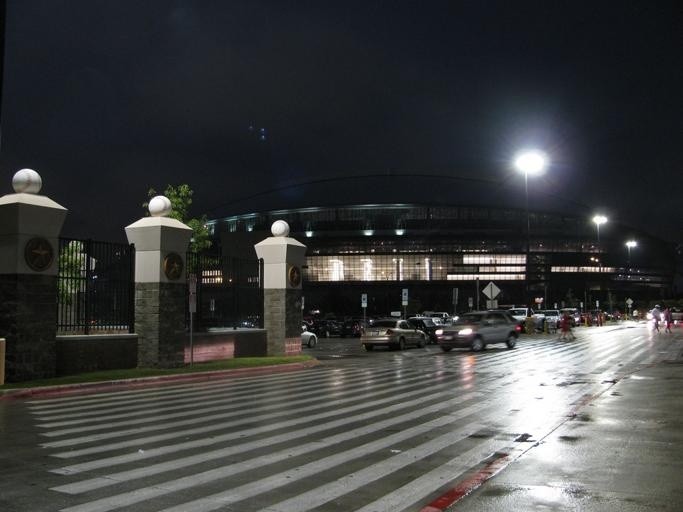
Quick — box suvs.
[434,311,521,353]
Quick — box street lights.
[626,240,637,321]
[516,146,547,336]
[593,215,608,325]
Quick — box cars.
[301,329,318,348]
[484,304,683,339]
[240,311,460,339]
[360,319,427,351]
[397,316,452,345]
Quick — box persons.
[663,306,672,333]
[558,312,570,341]
[650,304,661,334]
[562,312,576,339]
